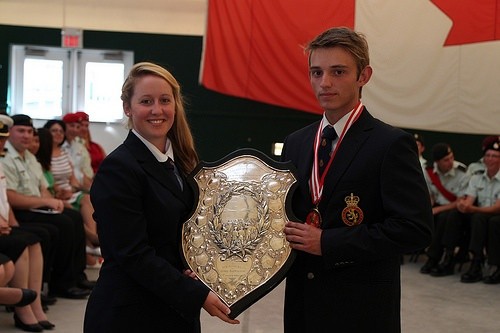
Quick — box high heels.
[13,311,56,332]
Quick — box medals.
[306,207,323,229]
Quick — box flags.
[198,0,500,137]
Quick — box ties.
[166,160,184,192]
[316,125,338,177]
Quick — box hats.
[63,113,81,123]
[431,142,453,161]
[413,133,423,143]
[0,115,14,136]
[484,139,500,152]
[76,111,89,122]
[9,114,34,127]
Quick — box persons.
[402,134,500,286]
[83,63,241,333]
[0,112,105,333]
[278,27,431,333]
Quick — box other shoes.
[461,262,484,282]
[86,256,104,268]
[484,267,500,285]
[42,295,57,312]
[420,253,442,273]
[0,288,37,308]
[50,279,97,298]
[432,251,455,277]
